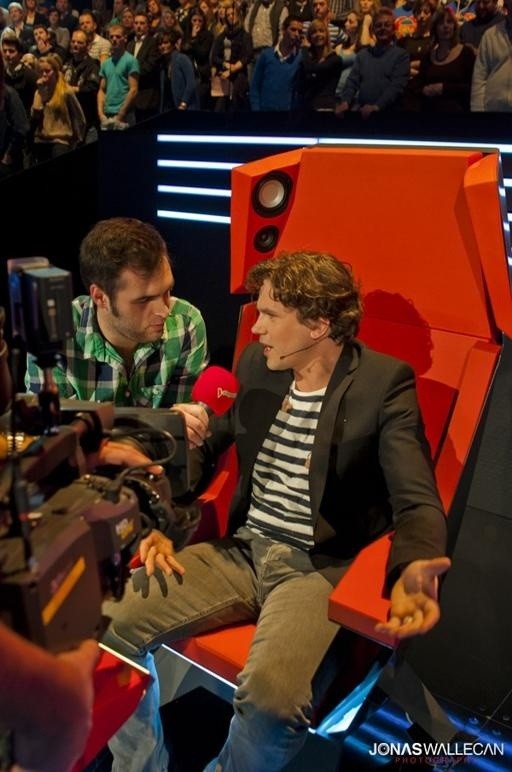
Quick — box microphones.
[280,332,333,359]
[189,364,242,419]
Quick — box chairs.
[68,144,512,771]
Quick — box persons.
[97,248,454,772]
[1,0,512,184]
[22,215,214,453]
